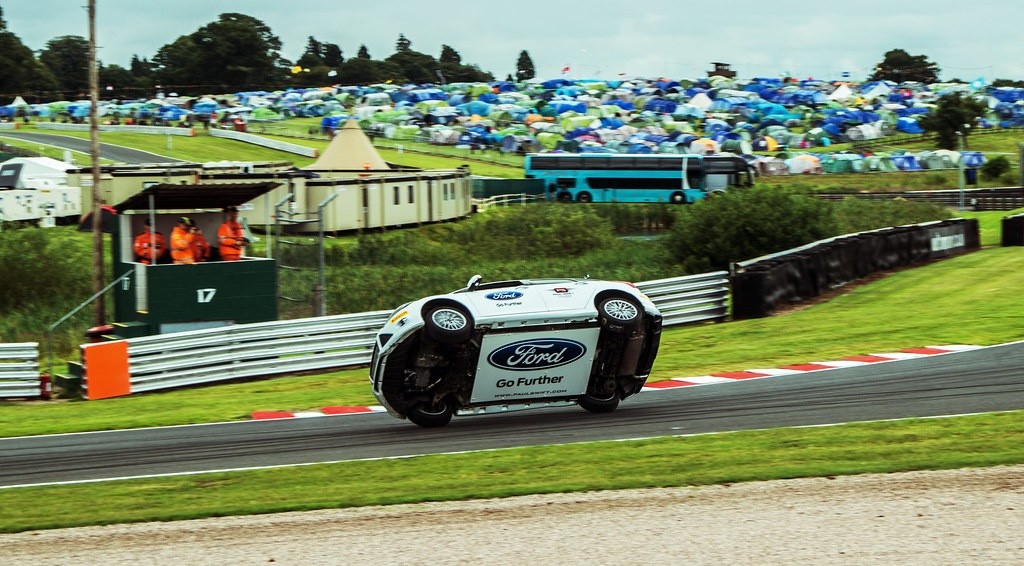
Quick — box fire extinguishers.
[40,368,52,399]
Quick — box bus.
[524,152,760,204]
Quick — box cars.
[368,273,663,429]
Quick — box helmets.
[177,217,196,227]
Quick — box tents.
[0,76,1023,181]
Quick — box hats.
[144,219,151,226]
[224,206,239,213]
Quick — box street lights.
[956,117,983,210]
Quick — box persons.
[216,207,251,261]
[964,164,979,189]
[133,220,167,264]
[170,217,211,264]
